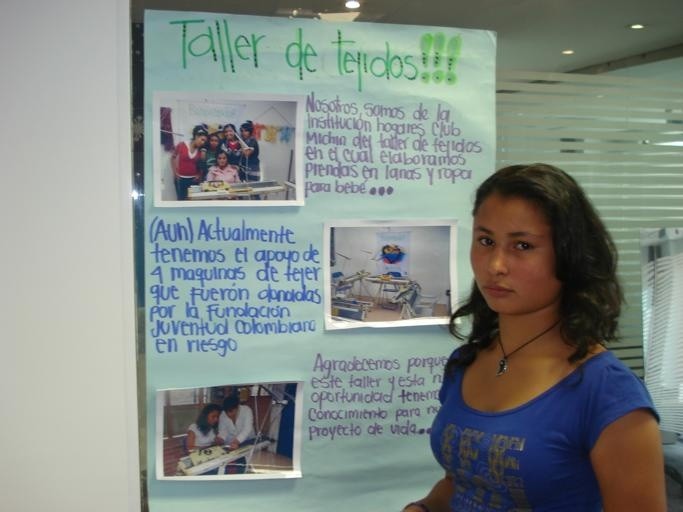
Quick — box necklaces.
[493,317,569,377]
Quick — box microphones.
[272,400,288,405]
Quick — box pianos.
[340,271,410,284]
[178,436,275,476]
[188,179,285,200]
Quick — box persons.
[183,394,255,474]
[171,119,259,201]
[401,164,669,512]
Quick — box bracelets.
[402,499,435,512]
